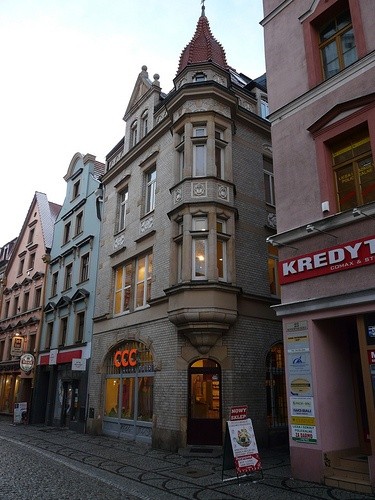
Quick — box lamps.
[264,207,375,252]
